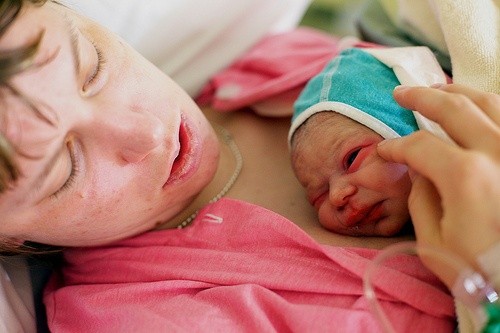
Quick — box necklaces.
[173,119,244,230]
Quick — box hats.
[287,48,419,150]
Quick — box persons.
[290,48,420,236]
[0,0,500,333]
